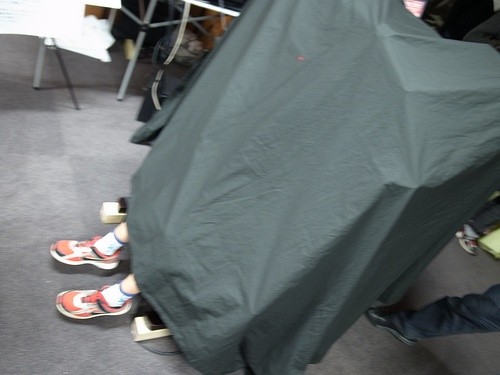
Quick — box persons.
[50,220,141,319]
[456,195,500,256]
[366,284,500,345]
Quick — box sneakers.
[56,285,132,320]
[365,307,420,346]
[456,231,477,255]
[50,236,121,270]
